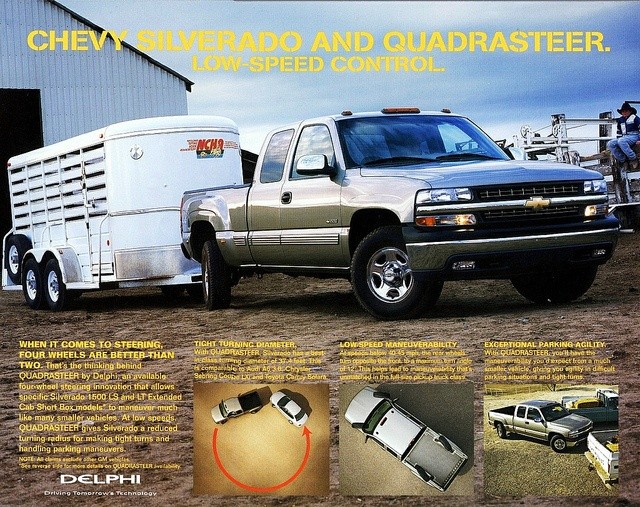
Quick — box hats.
[617,103,637,115]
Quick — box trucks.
[584,430,619,490]
[561,388,618,422]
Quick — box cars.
[270,391,308,428]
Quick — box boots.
[630,158,638,169]
[621,161,629,171]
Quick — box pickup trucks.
[487,399,594,452]
[344,386,469,492]
[179,108,622,321]
[211,391,262,424]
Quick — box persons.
[607,103,640,172]
